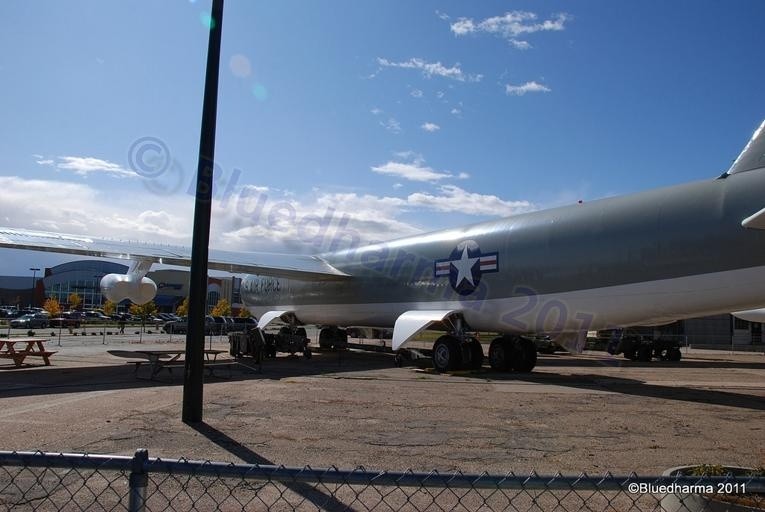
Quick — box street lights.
[28,267,42,308]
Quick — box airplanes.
[2,114,764,382]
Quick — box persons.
[119,315,126,334]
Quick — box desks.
[0,338,53,367]
[135,349,230,379]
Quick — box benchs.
[1,349,59,366]
[127,359,185,371]
[161,359,239,380]
[0,353,26,368]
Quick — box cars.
[0,303,260,336]
